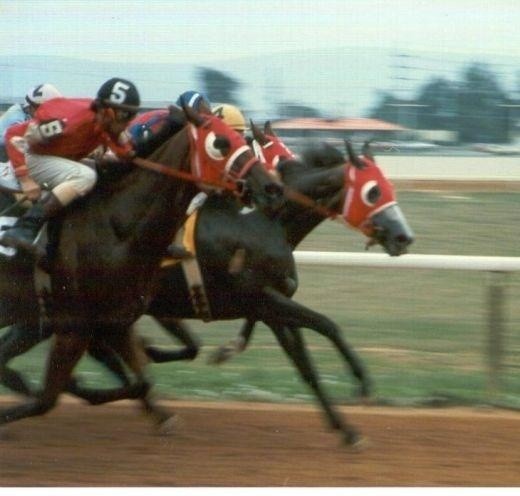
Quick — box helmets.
[98,78,139,108]
[25,83,61,106]
[176,91,250,130]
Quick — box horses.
[0,94,416,452]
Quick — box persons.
[165,103,248,258]
[0,77,140,265]
[105,91,212,160]
[1,82,61,145]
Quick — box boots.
[0,202,49,258]
